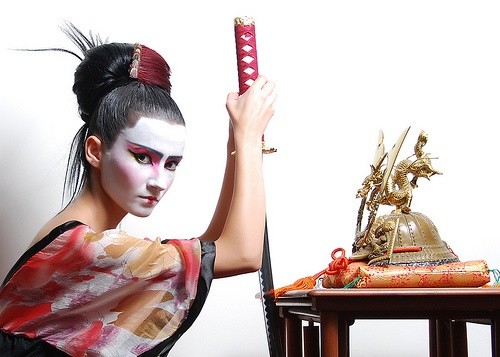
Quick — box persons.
[0,22,277,357]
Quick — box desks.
[257,288,500,357]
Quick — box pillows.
[272,247,500,289]
